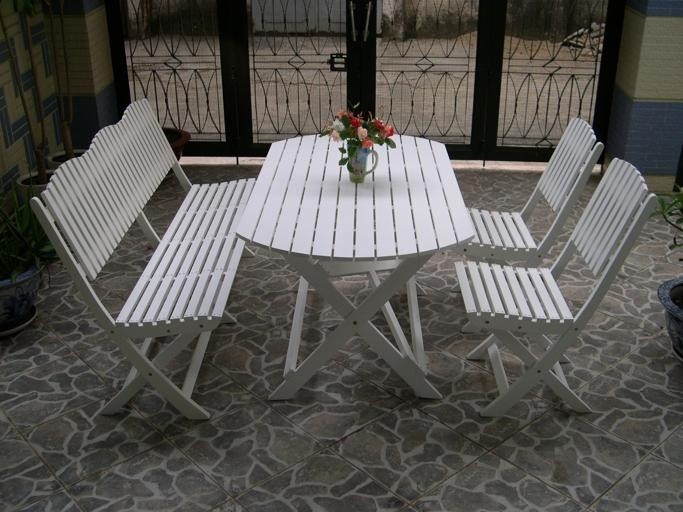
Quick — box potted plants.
[1,190,43,340]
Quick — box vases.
[15,126,191,220]
[648,179,683,361]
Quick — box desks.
[235,134,477,401]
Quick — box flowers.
[318,102,397,174]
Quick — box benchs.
[29,97,258,421]
